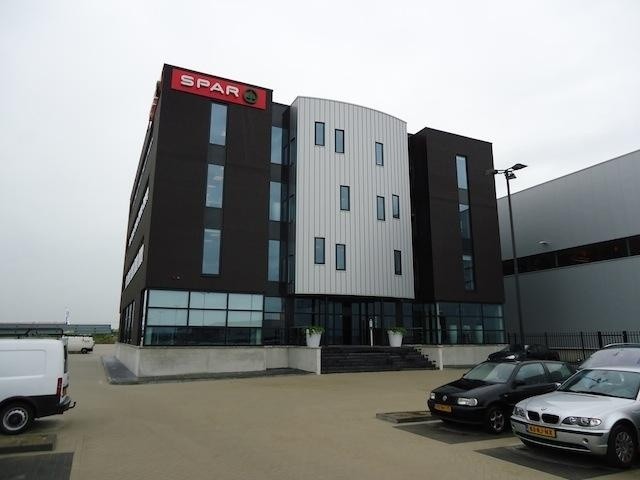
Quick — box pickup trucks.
[20,328,95,355]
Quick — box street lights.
[485,162,528,349]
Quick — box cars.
[514,342,640,469]
[0,335,77,435]
[424,346,576,435]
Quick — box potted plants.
[300,323,325,347]
[384,327,408,347]
